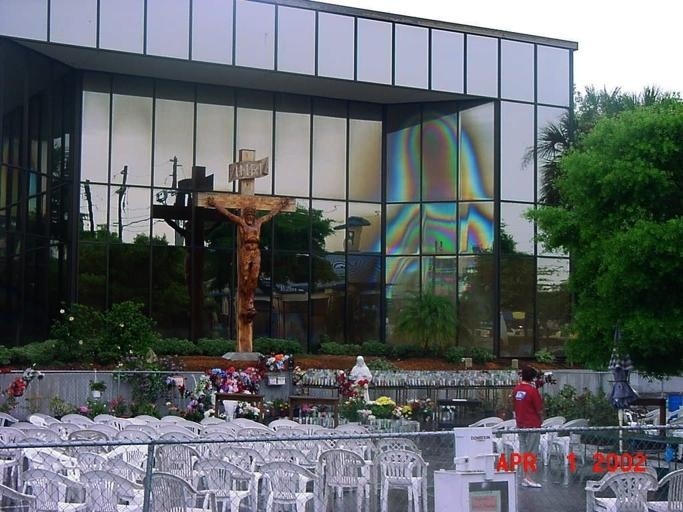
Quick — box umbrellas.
[607,326,641,453]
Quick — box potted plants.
[88,381,107,398]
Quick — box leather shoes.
[521,482,529,487]
[524,478,541,488]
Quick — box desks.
[436,398,482,427]
[214,393,265,424]
[288,395,340,428]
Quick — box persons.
[511,366,544,489]
[349,355,372,402]
[205,194,289,324]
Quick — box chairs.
[20,467,87,512]
[645,468,682,512]
[378,450,429,511]
[198,458,258,511]
[467,414,589,487]
[584,465,659,512]
[1,483,38,511]
[76,470,143,511]
[0,411,421,498]
[142,472,201,510]
[318,449,370,511]
[259,460,318,511]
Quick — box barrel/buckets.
[668,391,683,412]
